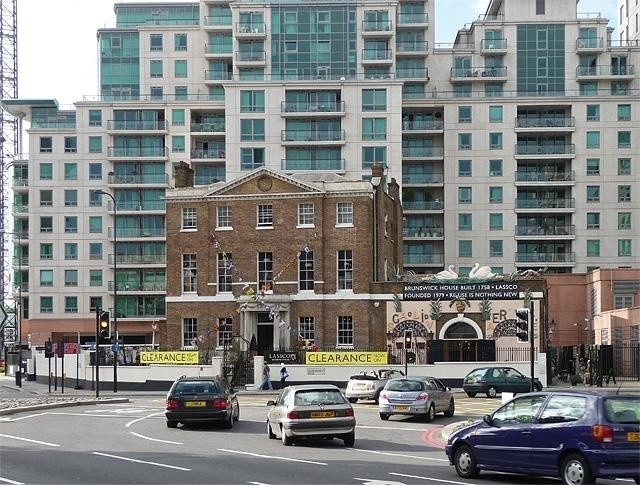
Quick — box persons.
[257,361,273,391]
[279,362,286,391]
[552,354,592,386]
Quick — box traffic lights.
[515,308,531,344]
[98,311,108,337]
[45,341,54,358]
[405,331,412,348]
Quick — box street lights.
[93,189,118,392]
[574,323,583,345]
[151,321,158,349]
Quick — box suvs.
[166,374,240,430]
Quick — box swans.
[469,263,496,279]
[434,265,458,280]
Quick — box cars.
[345,369,406,406]
[463,366,543,398]
[446,389,640,485]
[378,375,457,421]
[265,385,357,449]
